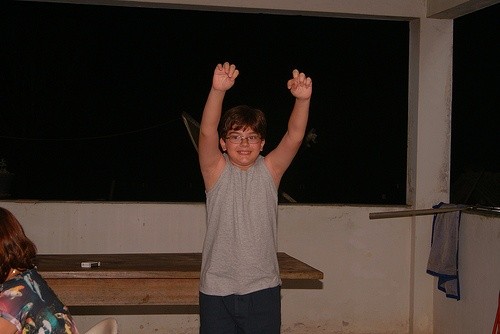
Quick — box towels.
[426,201,461,301]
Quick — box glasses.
[226,134,263,145]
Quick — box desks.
[33,252,323,306]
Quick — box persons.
[197,61,313,334]
[0,206,82,333]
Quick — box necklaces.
[5,268,17,281]
